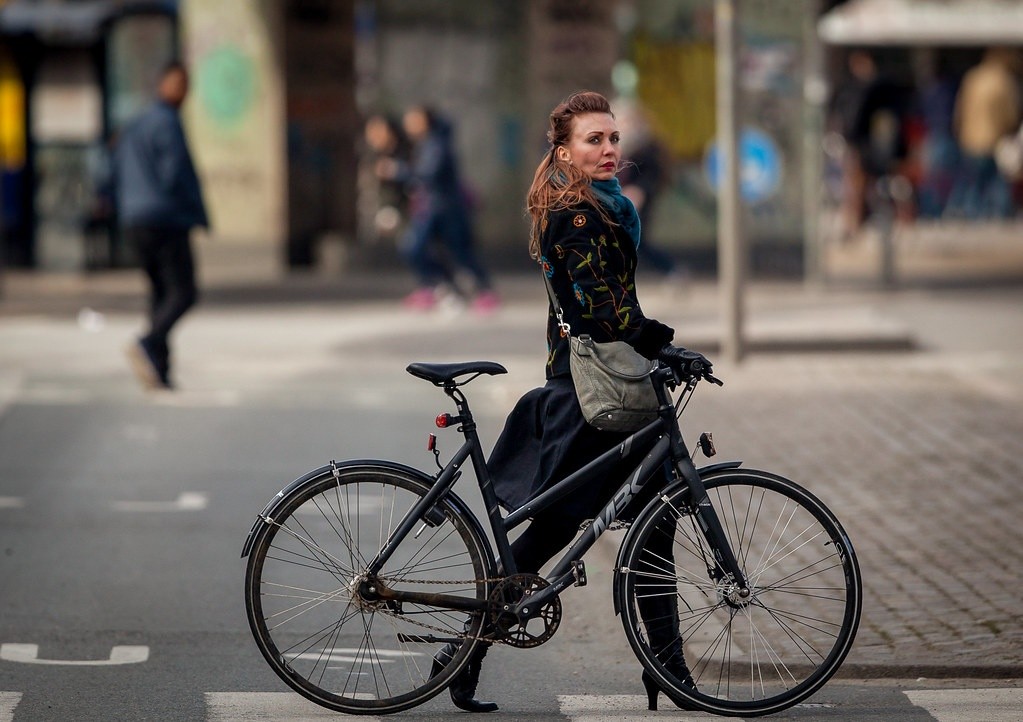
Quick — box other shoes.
[405,290,441,308]
[132,333,169,388]
[471,289,501,313]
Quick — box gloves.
[656,342,715,384]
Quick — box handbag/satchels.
[561,326,678,431]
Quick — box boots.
[634,556,713,712]
[421,556,541,713]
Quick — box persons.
[428,94,714,712]
[614,99,682,277]
[116,61,210,387]
[820,49,1023,223]
[1,50,26,262]
[368,105,500,310]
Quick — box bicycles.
[240,341,864,717]
